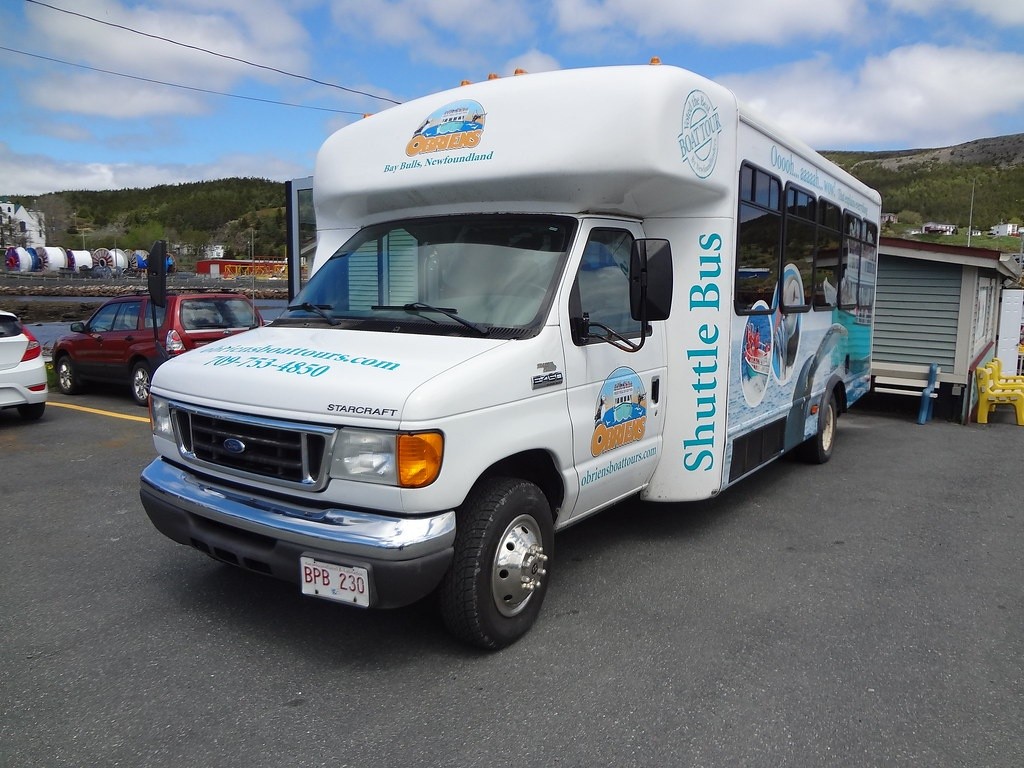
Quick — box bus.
[140,57,881,654]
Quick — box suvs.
[51,289,266,407]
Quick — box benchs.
[976,364,1024,426]
[988,358,1024,384]
[871,362,941,425]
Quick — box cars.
[0,310,48,420]
